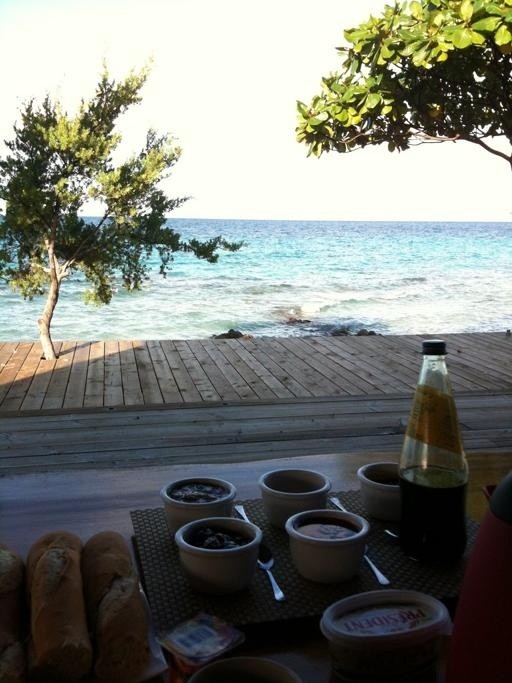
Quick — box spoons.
[255,548,286,604]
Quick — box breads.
[79,531,150,683]
[25,531,96,682]
[0,543,29,683]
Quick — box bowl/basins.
[160,476,239,547]
[257,468,334,530]
[184,656,305,681]
[172,516,264,589]
[285,509,372,583]
[357,461,404,525]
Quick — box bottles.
[397,337,470,567]
